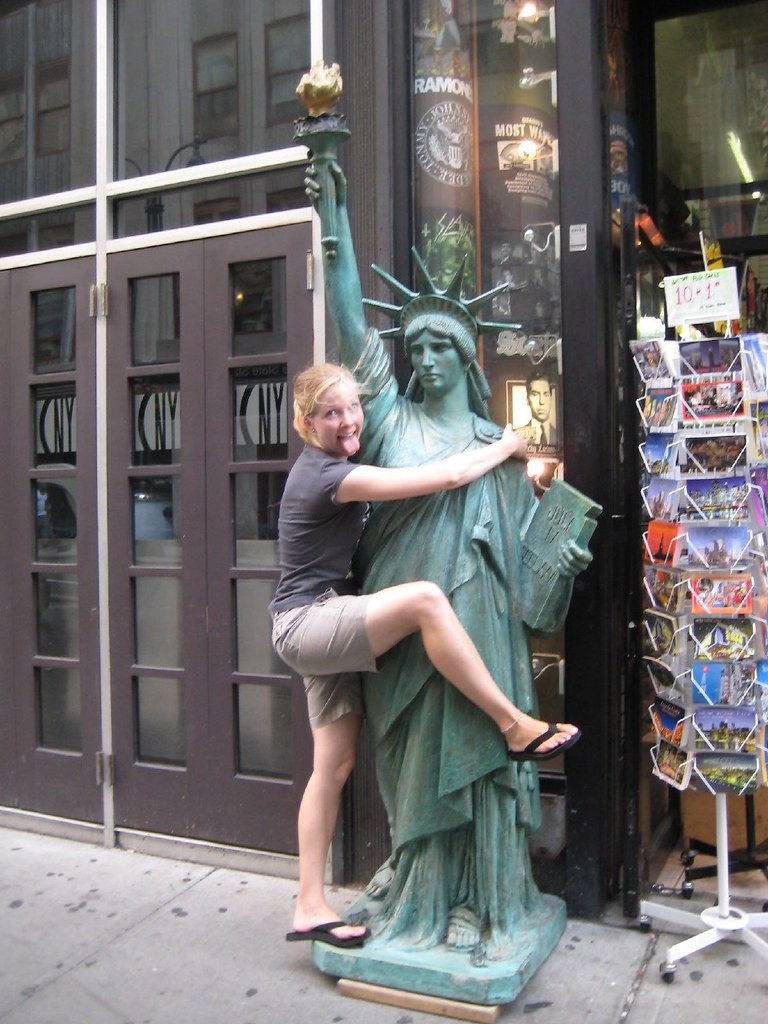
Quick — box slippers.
[509,723,581,759]
[286,922,371,947]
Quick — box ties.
[540,425,547,444]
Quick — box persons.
[513,372,558,453]
[492,241,525,294]
[304,150,594,961]
[268,363,582,947]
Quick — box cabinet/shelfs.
[630,333,768,983]
